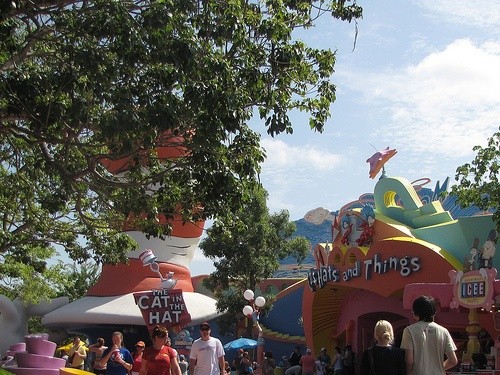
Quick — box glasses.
[201,328,209,331]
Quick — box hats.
[135,341,145,348]
[321,347,325,351]
[306,348,311,352]
[237,348,243,353]
[199,322,211,327]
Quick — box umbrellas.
[224,336,258,351]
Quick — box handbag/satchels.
[68,346,80,365]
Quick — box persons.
[53,321,225,375]
[400,296,458,375]
[225,344,354,375]
[359,320,406,375]
